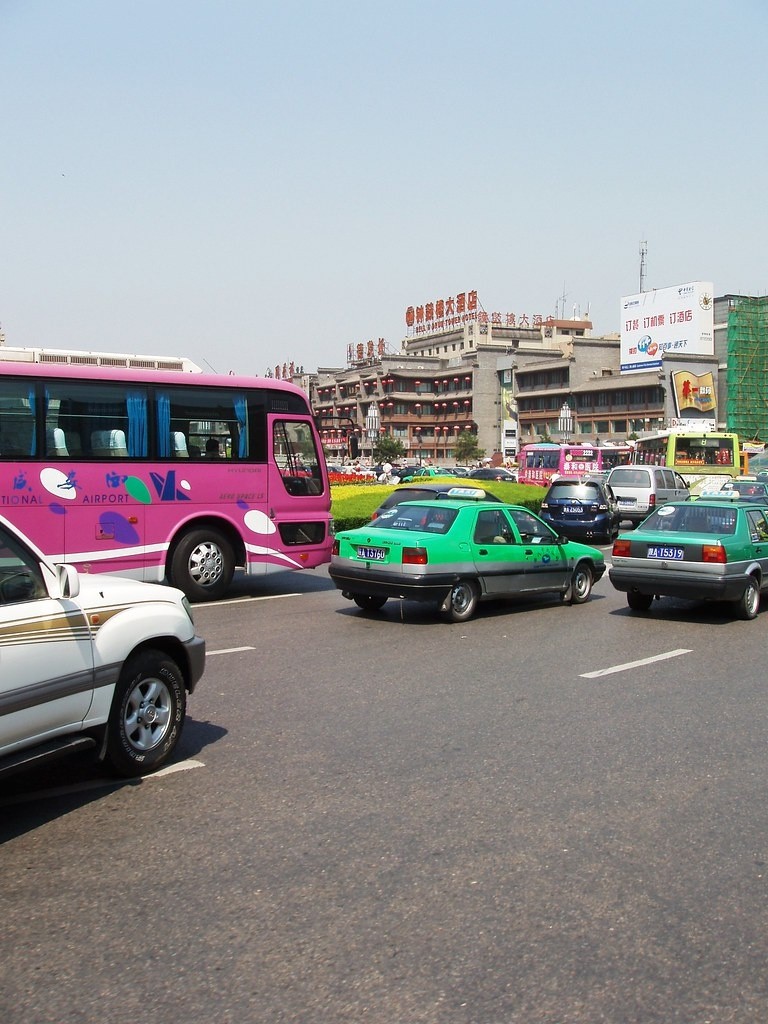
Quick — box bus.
[634,429,741,493]
[515,442,560,488]
[0,348,358,606]
[560,444,634,478]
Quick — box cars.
[608,490,768,619]
[366,482,537,540]
[536,476,621,545]
[325,454,514,485]
[327,487,608,626]
[704,466,768,527]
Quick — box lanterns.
[314,377,471,434]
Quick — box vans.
[604,463,693,528]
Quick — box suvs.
[0,512,207,785]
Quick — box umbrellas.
[484,457,492,463]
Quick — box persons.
[343,454,510,476]
[203,439,221,458]
[548,468,563,483]
